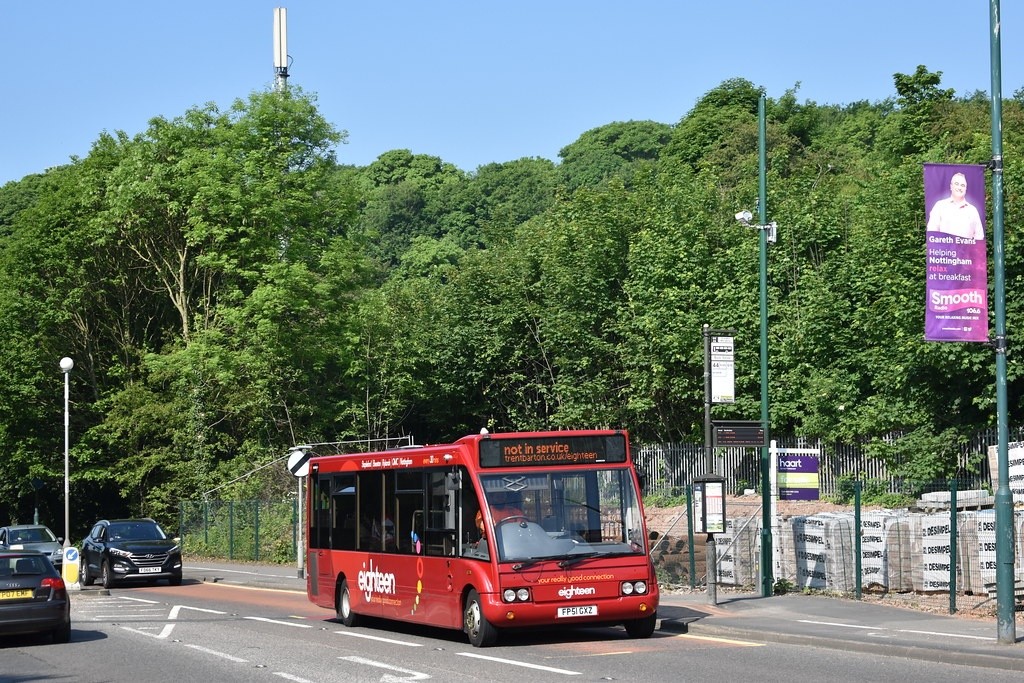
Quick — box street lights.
[59,357,74,550]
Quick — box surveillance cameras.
[734,211,752,222]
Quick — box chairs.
[15,560,34,572]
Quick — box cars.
[0,549,72,645]
[0,524,64,575]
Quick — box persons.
[924,172,984,240]
[475,490,524,539]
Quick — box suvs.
[80,518,183,589]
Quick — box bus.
[305,427,661,648]
